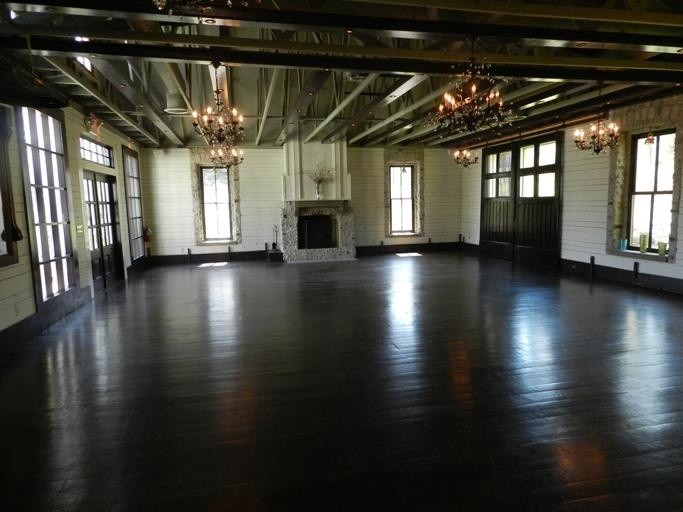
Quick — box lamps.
[423,33,528,171]
[644,125,655,145]
[572,79,633,156]
[189,58,246,171]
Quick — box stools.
[267,250,283,264]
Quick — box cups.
[657,242,666,256]
[619,238,627,252]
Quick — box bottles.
[640,232,648,252]
